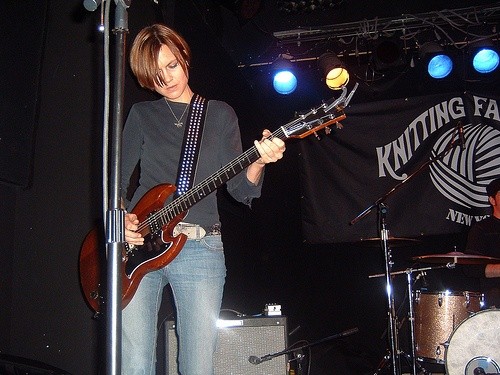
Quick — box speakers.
[164,317,289,375]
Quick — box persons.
[465,179,500,309]
[118,25,286,375]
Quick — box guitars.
[79,80,359,315]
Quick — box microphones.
[458,121,466,151]
[249,356,260,365]
[84,0,101,11]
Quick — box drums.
[411,287,488,365]
[442,306,500,375]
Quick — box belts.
[172,222,221,240]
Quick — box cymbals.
[412,251,500,265]
[360,237,420,248]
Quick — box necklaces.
[165,93,194,128]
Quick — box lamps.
[269,50,349,95]
[419,40,500,79]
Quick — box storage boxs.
[164,316,289,375]
